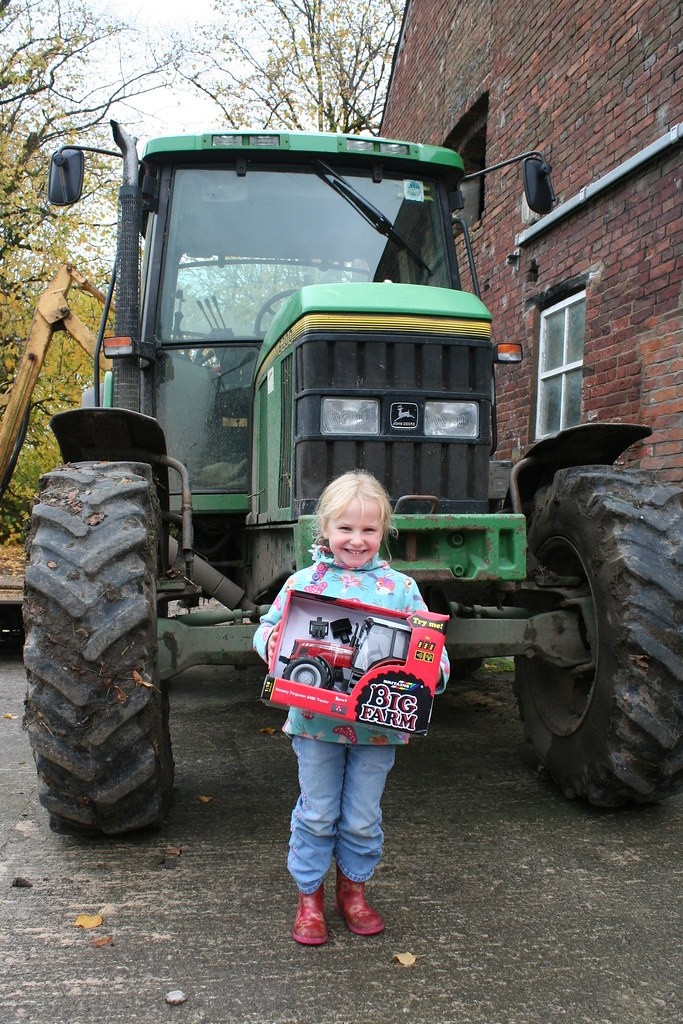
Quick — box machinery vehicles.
[22,120,683,841]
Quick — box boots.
[291,880,327,945]
[335,864,384,935]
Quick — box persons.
[253,472,450,943]
